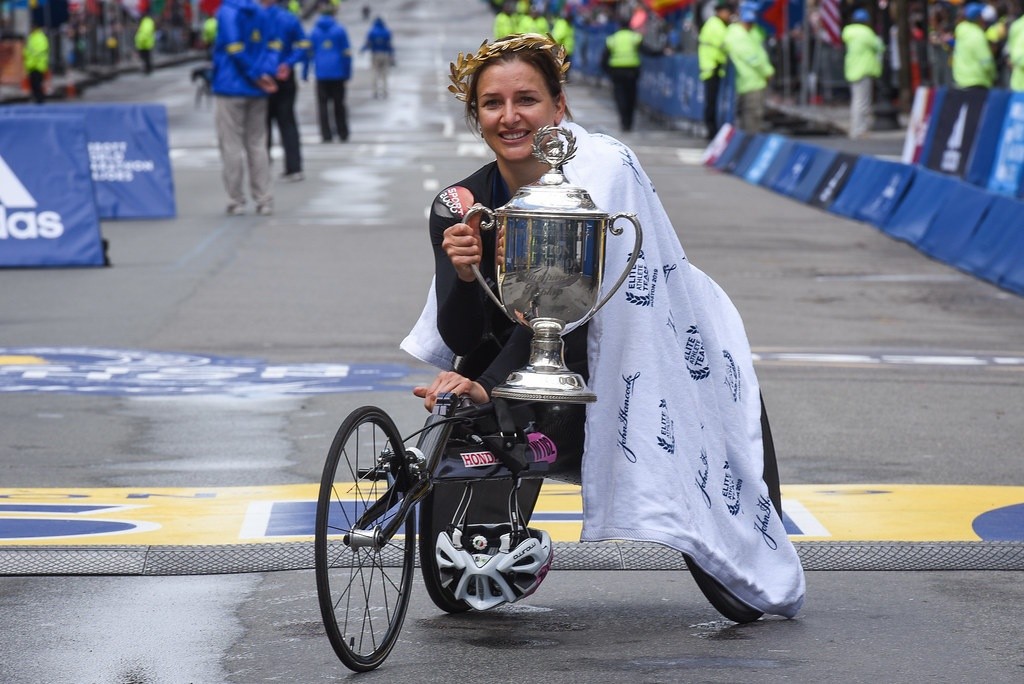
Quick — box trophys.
[462,126,643,404]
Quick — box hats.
[851,9,871,24]
[742,8,756,24]
[965,4,983,22]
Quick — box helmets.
[436,520,555,612]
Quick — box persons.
[400,33,805,618]
[22,0,191,104]
[201,1,394,215]
[494,0,1024,142]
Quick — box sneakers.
[225,201,245,214]
[258,197,274,214]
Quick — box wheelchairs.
[312,332,784,675]
